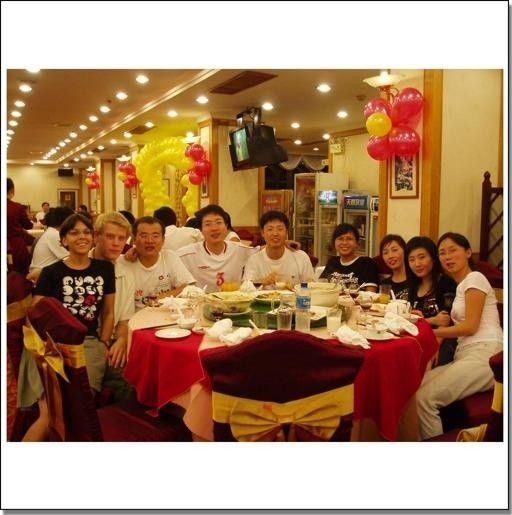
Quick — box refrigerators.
[318,189,343,266]
[342,189,378,258]
[293,171,350,257]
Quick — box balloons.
[84,134,214,222]
[363,86,425,161]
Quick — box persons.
[235,135,244,163]
[320,224,379,293]
[7,177,242,285]
[240,209,316,288]
[379,233,410,302]
[413,230,503,442]
[26,211,136,402]
[406,236,457,369]
[113,216,200,315]
[122,204,302,293]
[17,213,117,442]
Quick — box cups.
[253,311,267,334]
[276,309,292,330]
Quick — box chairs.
[5,269,186,442]
[199,329,362,442]
[417,351,505,442]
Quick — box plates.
[222,308,252,317]
[142,284,203,341]
[250,289,293,304]
[339,283,420,341]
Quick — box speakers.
[58,168,73,176]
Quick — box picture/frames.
[201,176,209,199]
[389,148,420,201]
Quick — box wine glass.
[220,275,285,290]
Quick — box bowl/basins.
[206,291,253,313]
[294,281,342,306]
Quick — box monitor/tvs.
[228,121,288,172]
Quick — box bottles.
[293,283,312,333]
